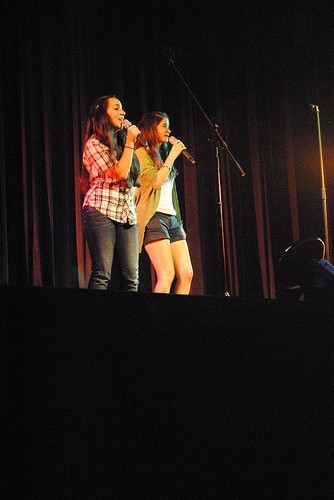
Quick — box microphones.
[121,119,149,150]
[168,136,195,164]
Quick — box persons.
[80,94,142,293]
[136,111,194,294]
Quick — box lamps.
[276,236,334,305]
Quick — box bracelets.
[163,164,172,171]
[124,145,134,149]
